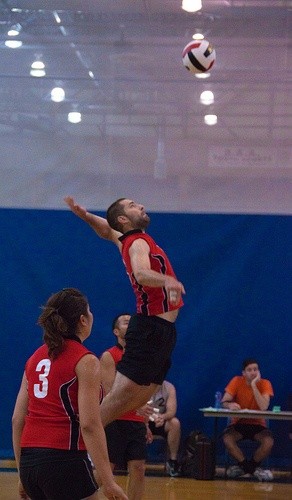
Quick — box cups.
[272,402,281,413]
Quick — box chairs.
[224,416,270,477]
[152,436,170,475]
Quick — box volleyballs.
[182,40,216,74]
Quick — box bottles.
[215,391,222,409]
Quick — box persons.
[88,313,147,500]
[218,361,277,483]
[139,369,184,479]
[60,190,186,440]
[9,286,133,500]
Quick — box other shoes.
[253,467,274,482]
[226,464,244,478]
[166,458,180,476]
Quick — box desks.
[200,408,292,480]
